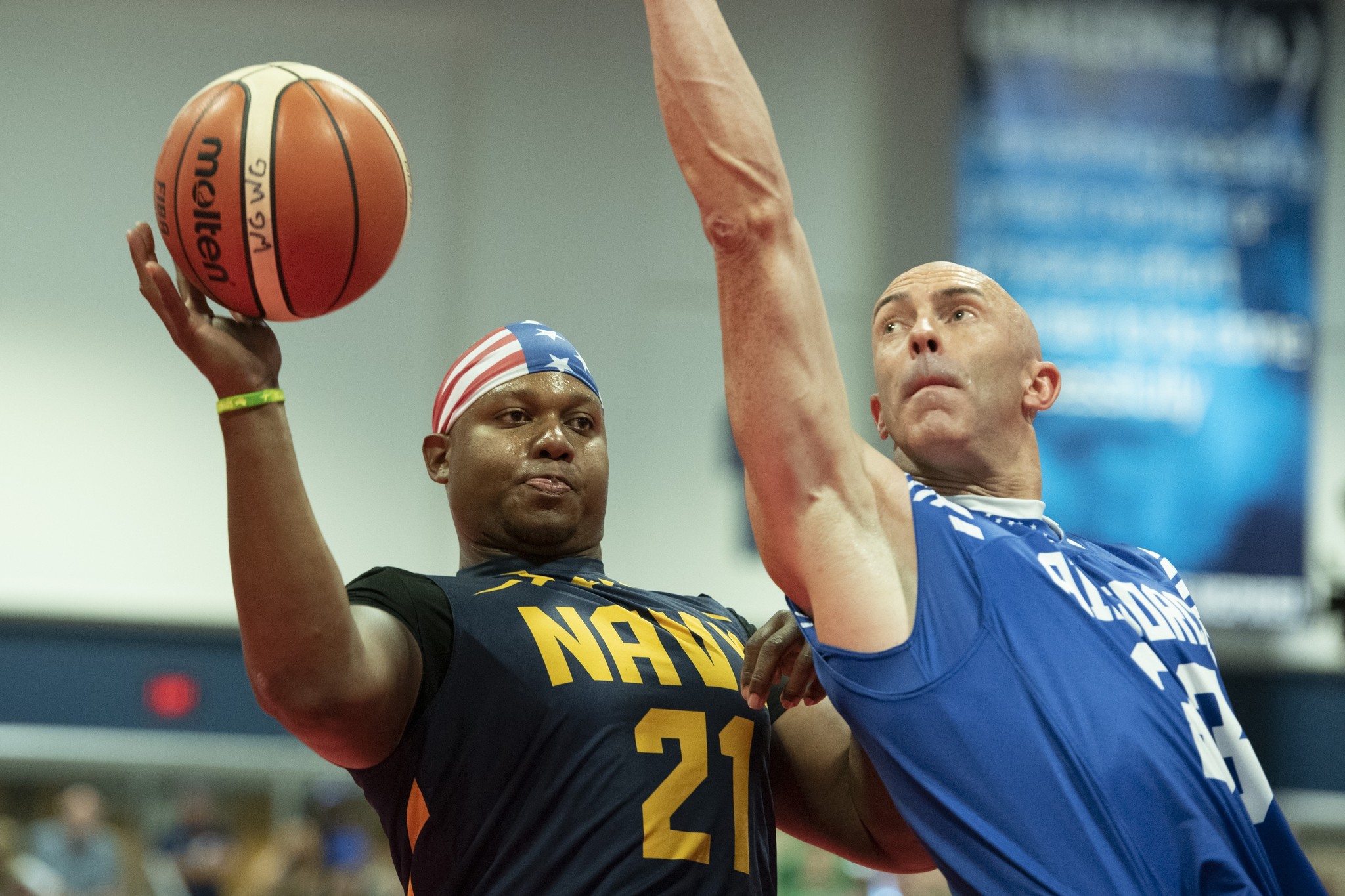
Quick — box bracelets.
[215,388,289,416]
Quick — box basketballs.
[153,60,413,323]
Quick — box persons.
[124,223,938,896]
[1,750,405,896]
[638,1,1324,896]
